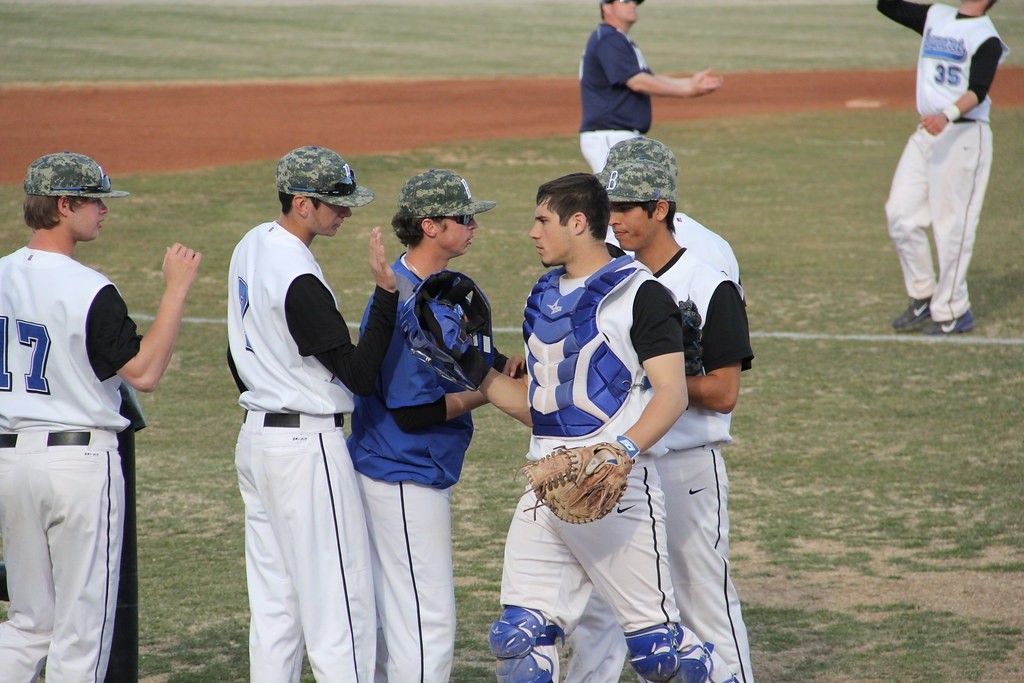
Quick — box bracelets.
[943,105,960,122]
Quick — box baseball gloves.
[522,442,634,523]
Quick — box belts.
[243,408,345,429]
[953,116,973,123]
[1,429,92,446]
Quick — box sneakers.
[892,295,934,328]
[924,307,974,336]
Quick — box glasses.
[444,216,474,227]
[48,173,114,196]
[286,168,357,198]
[603,0,637,6]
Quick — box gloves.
[417,274,491,386]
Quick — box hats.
[398,167,496,218]
[595,138,679,185]
[598,158,677,205]
[23,153,129,198]
[275,143,375,206]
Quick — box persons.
[578,0,724,173]
[0,150,202,683]
[398,171,741,683]
[503,133,754,683]
[226,145,401,683]
[346,169,529,683]
[876,0,1010,338]
